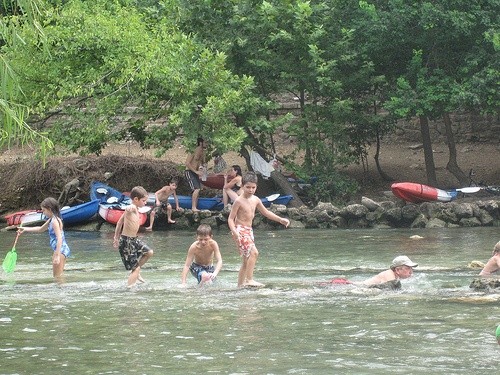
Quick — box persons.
[144,177,180,232]
[228,173,290,288]
[180,225,223,289]
[479,241,500,275]
[352,256,418,291]
[185,138,208,211]
[112,186,153,287]
[16,197,70,282]
[223,165,244,207]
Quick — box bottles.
[202,166,207,181]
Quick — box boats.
[5,200,101,230]
[202,173,317,189]
[124,192,292,210]
[90,178,148,225]
[391,182,457,202]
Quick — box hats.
[390,255,418,271]
